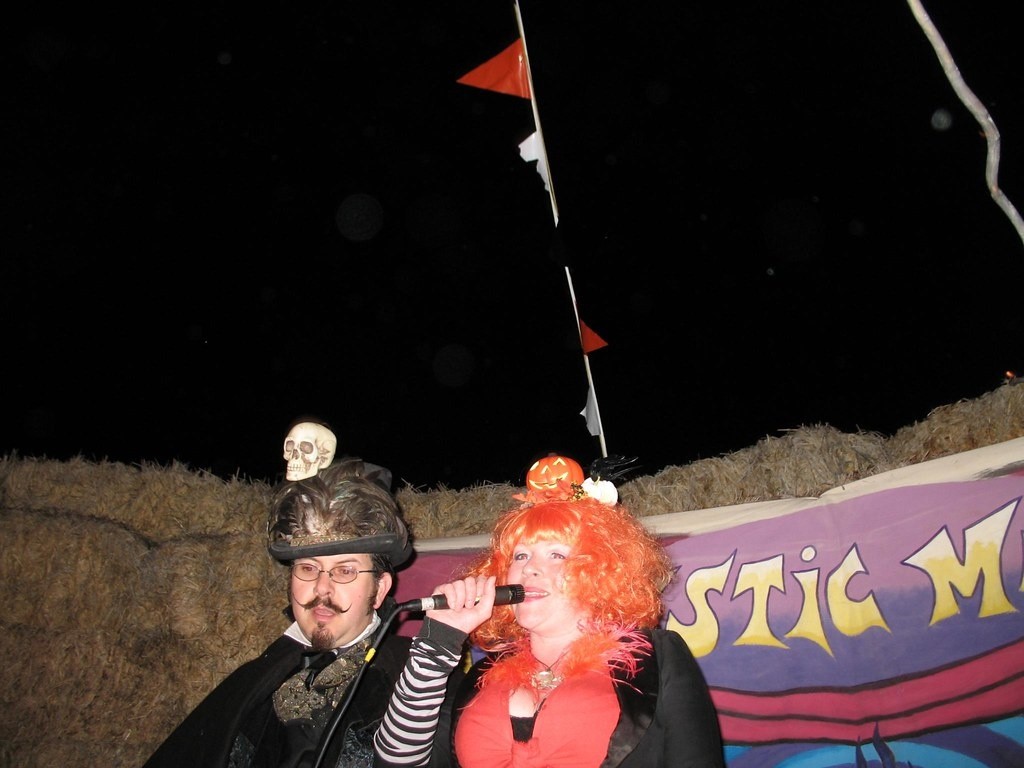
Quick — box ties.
[305,649,336,693]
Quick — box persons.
[373,497,725,768]
[141,463,466,768]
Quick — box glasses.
[290,563,386,585]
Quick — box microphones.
[404,584,525,611]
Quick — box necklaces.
[528,653,570,686]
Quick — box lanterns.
[526,455,585,498]
